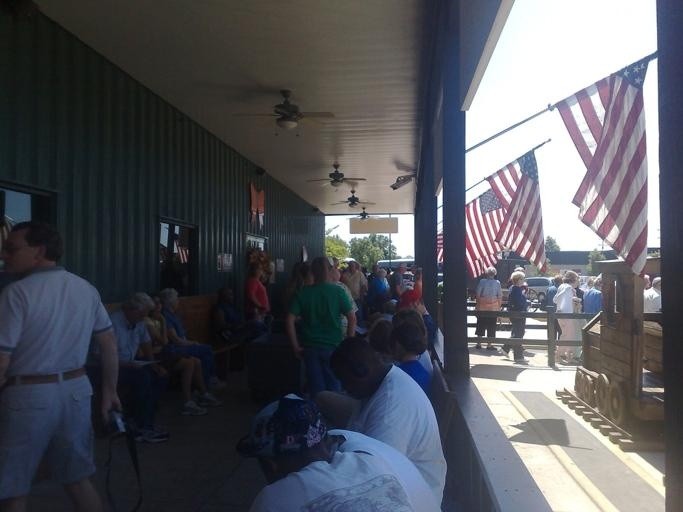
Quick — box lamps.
[329,181,342,189]
[347,203,358,208]
[359,218,368,222]
[273,118,298,131]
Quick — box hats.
[235,392,327,456]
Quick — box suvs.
[519,274,566,306]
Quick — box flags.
[555,57,652,280]
[464,186,506,280]
[484,150,548,275]
[435,229,443,263]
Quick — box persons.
[474,266,502,352]
[506,266,661,365]
[0,218,123,510]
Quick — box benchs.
[97,294,237,424]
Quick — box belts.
[6,368,88,385]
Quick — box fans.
[329,189,376,208]
[306,162,366,189]
[227,87,335,130]
[342,206,389,222]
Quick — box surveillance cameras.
[390,175,411,190]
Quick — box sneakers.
[134,426,171,444]
[486,345,498,351]
[177,399,208,417]
[198,391,225,409]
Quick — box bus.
[373,259,444,294]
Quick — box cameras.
[106,411,126,437]
